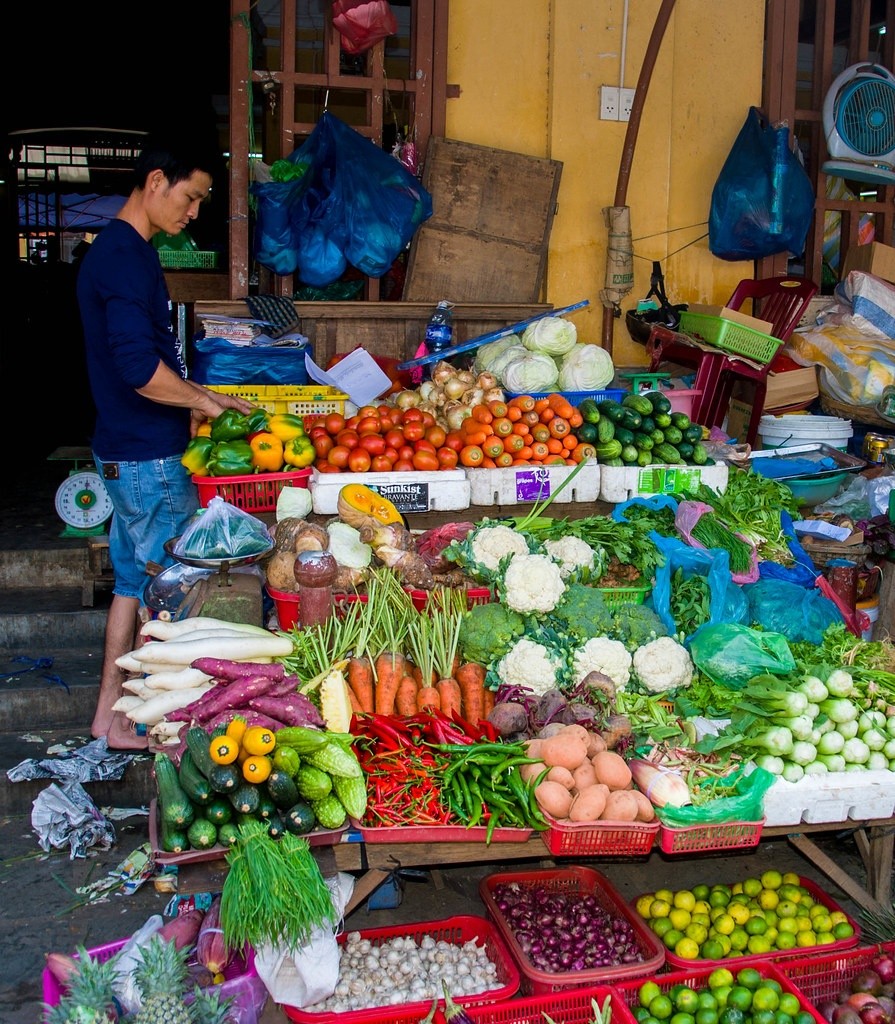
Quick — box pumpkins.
[268,482,404,557]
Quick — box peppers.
[344,707,552,847]
[179,406,315,510]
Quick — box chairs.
[639,274,819,453]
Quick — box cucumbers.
[576,392,715,467]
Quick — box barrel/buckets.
[852,593,878,646]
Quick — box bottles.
[417,300,456,386]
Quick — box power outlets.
[618,87,636,122]
[600,85,620,122]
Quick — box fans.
[819,60,895,187]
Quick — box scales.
[46,446,116,541]
[163,531,277,632]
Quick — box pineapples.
[36,932,246,1024]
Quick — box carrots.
[458,394,597,467]
[311,567,495,728]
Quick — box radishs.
[109,616,321,746]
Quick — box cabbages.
[472,316,615,394]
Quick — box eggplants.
[417,979,611,1024]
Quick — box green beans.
[659,741,759,827]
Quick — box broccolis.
[445,522,695,699]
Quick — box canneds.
[862,431,895,468]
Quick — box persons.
[75,122,261,760]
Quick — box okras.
[615,690,682,742]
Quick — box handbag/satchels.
[250,111,434,287]
[709,105,815,262]
[173,495,274,559]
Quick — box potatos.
[484,670,655,849]
[162,657,326,761]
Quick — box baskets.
[282,915,520,1024]
[429,984,639,1024]
[775,941,895,1007]
[191,465,314,514]
[42,935,261,1023]
[655,816,767,854]
[536,801,662,857]
[502,387,628,407]
[479,865,665,998]
[677,310,785,364]
[630,876,860,974]
[157,250,221,269]
[611,959,830,1024]
[199,384,349,423]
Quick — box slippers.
[106,746,156,756]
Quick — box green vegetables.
[694,470,895,783]
[509,455,692,579]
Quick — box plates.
[144,558,269,614]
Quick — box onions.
[490,882,647,977]
[395,361,505,433]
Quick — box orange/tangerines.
[630,871,854,1024]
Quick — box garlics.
[297,931,506,1013]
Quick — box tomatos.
[310,405,464,473]
